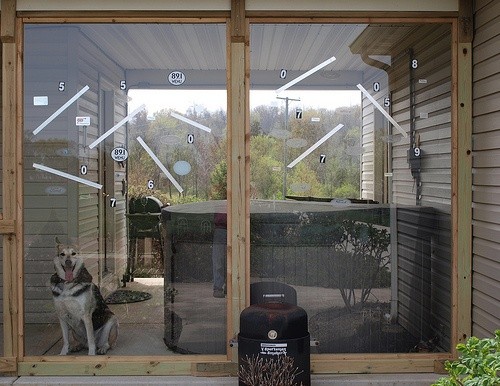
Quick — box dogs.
[48,237,119,356]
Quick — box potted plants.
[292,210,392,352]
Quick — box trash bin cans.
[237,303,311,386]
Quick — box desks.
[126,213,164,283]
